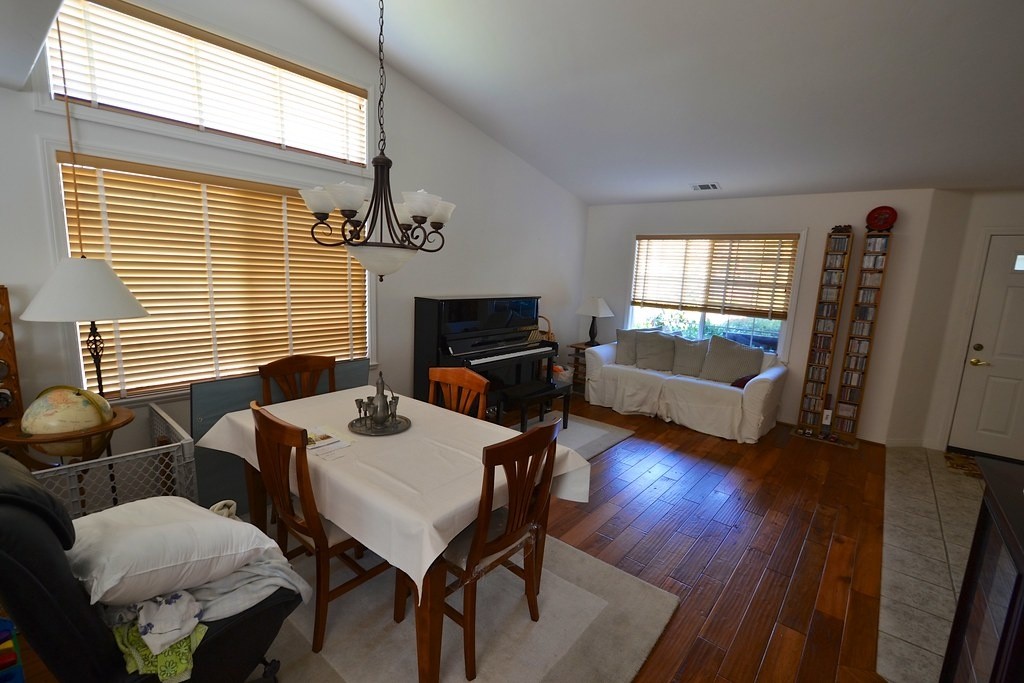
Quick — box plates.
[865,205,898,230]
[348,414,411,436]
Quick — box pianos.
[411,293,560,419]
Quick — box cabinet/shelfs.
[796,233,854,435]
[829,233,892,443]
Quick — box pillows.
[672,335,710,378]
[66,495,283,605]
[699,335,764,382]
[636,331,683,371]
[616,326,662,365]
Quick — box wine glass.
[356,396,401,432]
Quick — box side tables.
[568,342,611,391]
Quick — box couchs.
[584,341,789,445]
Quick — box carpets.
[944,451,984,479]
[509,410,636,461]
[244,501,682,683]
[789,427,860,449]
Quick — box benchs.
[497,381,571,433]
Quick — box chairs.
[1,454,303,683]
[259,354,336,406]
[250,401,393,653]
[394,417,562,682]
[428,367,490,420]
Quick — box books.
[833,237,888,433]
[800,235,849,425]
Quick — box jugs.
[370,371,394,429]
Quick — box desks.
[938,457,1024,683]
[195,385,591,683]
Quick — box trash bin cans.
[543,363,574,399]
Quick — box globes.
[0,385,136,516]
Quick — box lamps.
[19,255,149,507]
[575,297,614,346]
[299,1,456,283]
